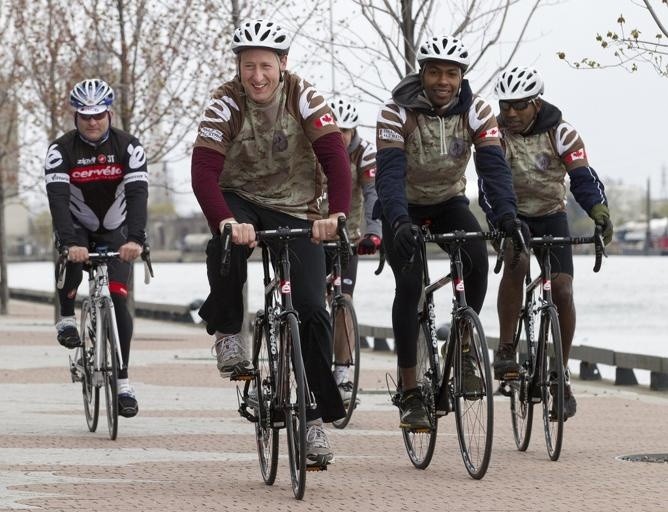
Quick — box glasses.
[78,113,107,120]
[499,101,529,111]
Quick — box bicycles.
[61,244,154,441]
[212,216,348,456]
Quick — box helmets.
[68,79,114,115]
[328,96,361,129]
[494,66,544,101]
[416,35,469,74]
[232,19,290,55]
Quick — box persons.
[312,94,383,411]
[44,79,149,416]
[372,34,521,433]
[191,20,354,467]
[477,66,613,416]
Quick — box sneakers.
[306,418,332,462]
[211,330,249,372]
[451,346,484,401]
[337,381,360,405]
[548,369,577,417]
[493,345,519,379]
[117,389,139,417]
[398,389,431,430]
[58,325,81,348]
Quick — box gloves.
[357,233,381,255]
[390,216,423,254]
[589,204,613,246]
[495,214,530,250]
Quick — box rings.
[327,233,332,235]
[132,255,137,260]
